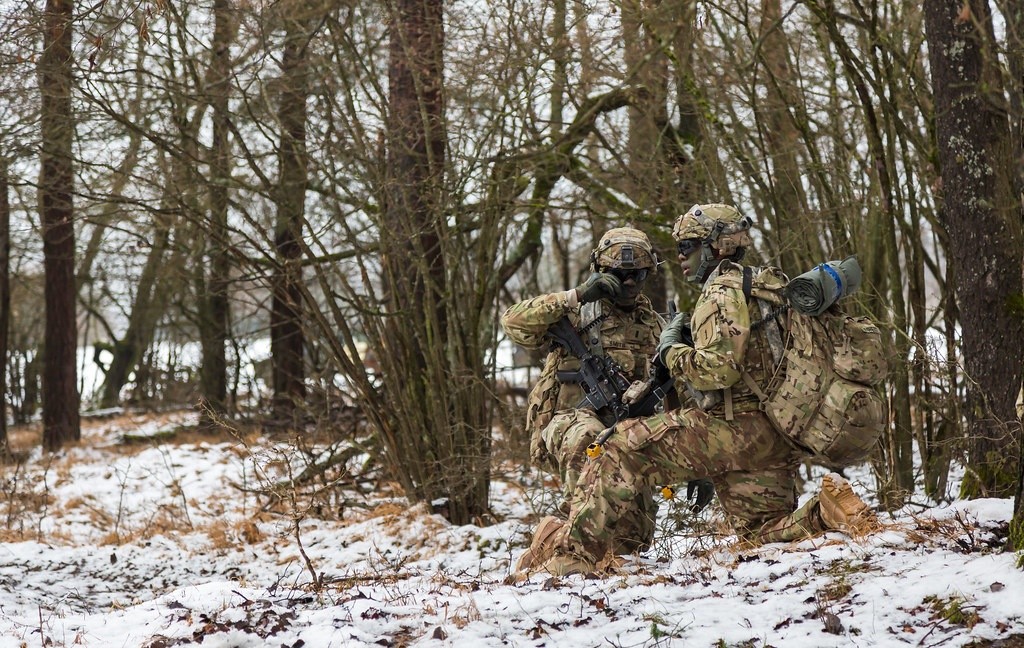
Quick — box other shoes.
[514,514,566,570]
[505,553,599,580]
[820,474,878,537]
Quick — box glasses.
[604,267,648,283]
[676,240,705,256]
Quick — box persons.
[505,203,881,586]
[500,227,715,556]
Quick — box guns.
[653,301,694,410]
[548,315,645,427]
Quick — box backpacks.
[524,349,555,464]
[712,266,887,465]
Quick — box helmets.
[591,228,659,310]
[671,204,754,283]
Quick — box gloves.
[659,313,686,368]
[576,273,623,302]
